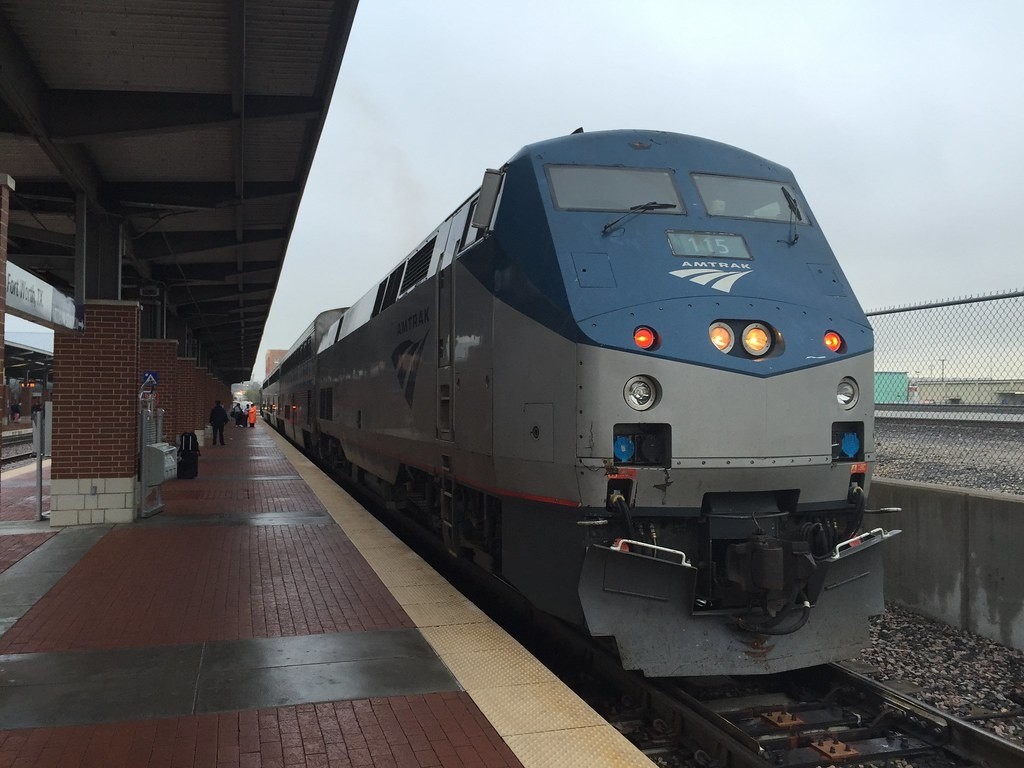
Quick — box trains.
[258,127,903,676]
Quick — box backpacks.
[180,431,198,452]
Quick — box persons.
[234,403,257,428]
[210,400,230,446]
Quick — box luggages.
[176,434,198,479]
